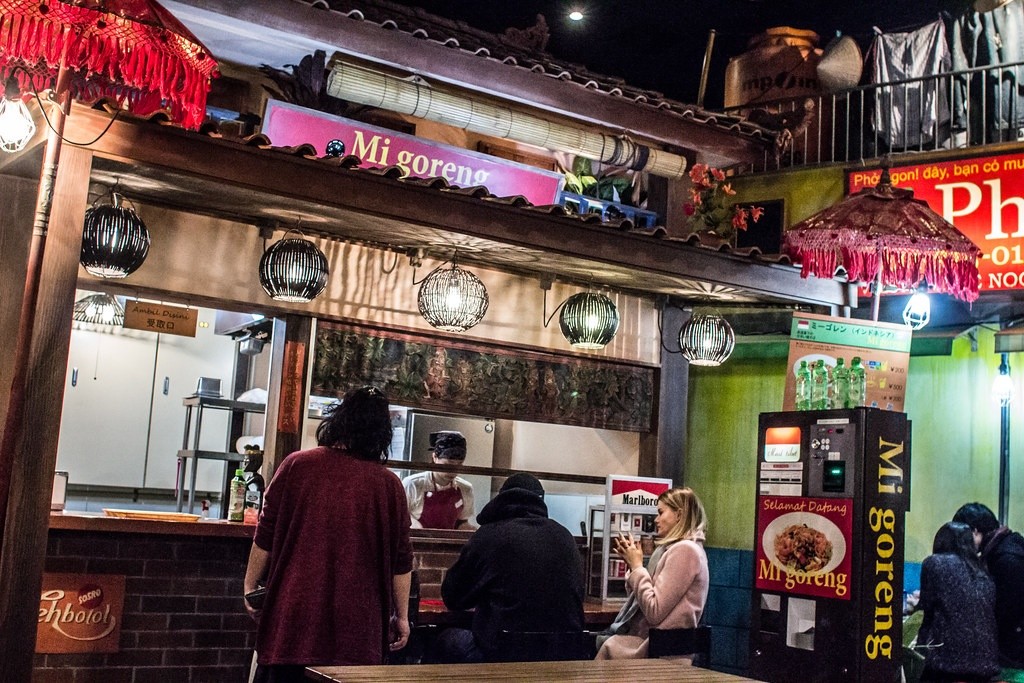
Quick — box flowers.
[683,163,763,231]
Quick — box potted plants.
[559,157,657,231]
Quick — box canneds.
[641,536,654,555]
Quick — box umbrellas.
[0,0,220,542]
[780,151,981,321]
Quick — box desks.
[409,594,624,628]
[303,658,763,683]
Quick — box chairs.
[498,628,591,662]
[648,626,712,669]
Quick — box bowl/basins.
[763,512,846,577]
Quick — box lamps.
[406,248,489,333]
[654,295,735,367]
[79,175,151,279]
[538,274,620,350]
[903,270,930,331]
[260,219,330,302]
[0,64,33,153]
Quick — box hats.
[499,472,545,502]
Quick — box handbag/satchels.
[244,586,267,610]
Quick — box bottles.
[832,358,850,409]
[850,356,865,409]
[795,360,811,411]
[811,360,829,410]
[228,469,246,520]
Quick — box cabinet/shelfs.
[585,506,662,605]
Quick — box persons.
[952,502,1024,669]
[434,472,587,664]
[243,387,415,683]
[589,488,710,661]
[902,522,1001,683]
[402,431,481,530]
